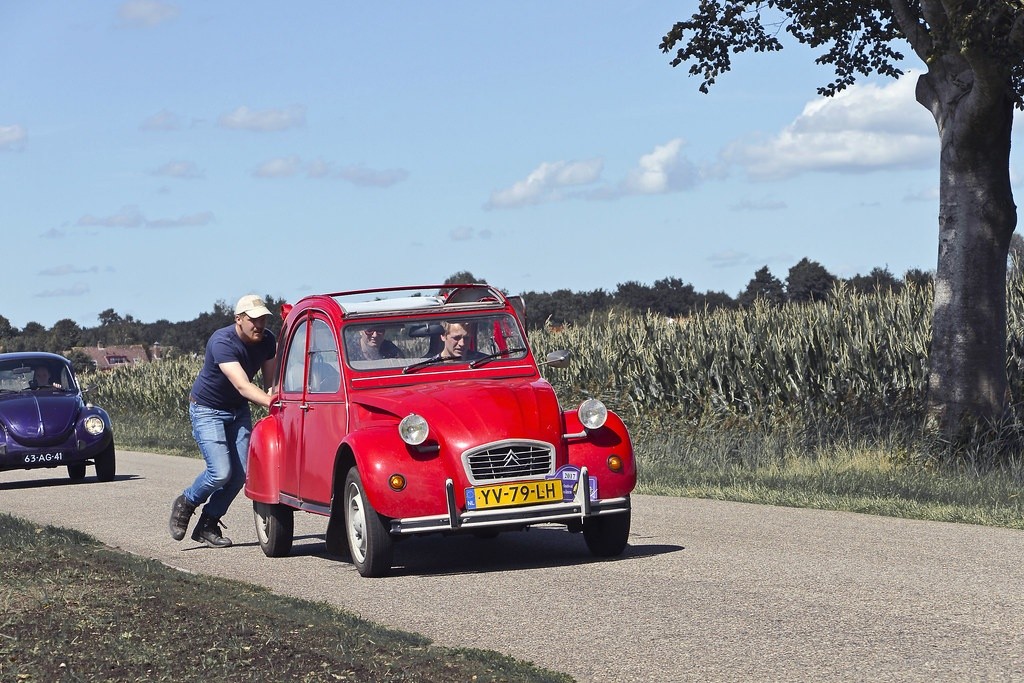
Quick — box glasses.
[364,328,385,335]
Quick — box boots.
[169,494,197,541]
[191,511,232,548]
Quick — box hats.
[234,294,274,319]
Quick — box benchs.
[289,358,435,392]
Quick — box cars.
[245,281,638,577]
[1,352,116,484]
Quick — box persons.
[169,294,280,548]
[348,327,405,361]
[425,321,492,364]
[32,366,62,394]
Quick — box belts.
[188,393,196,403]
